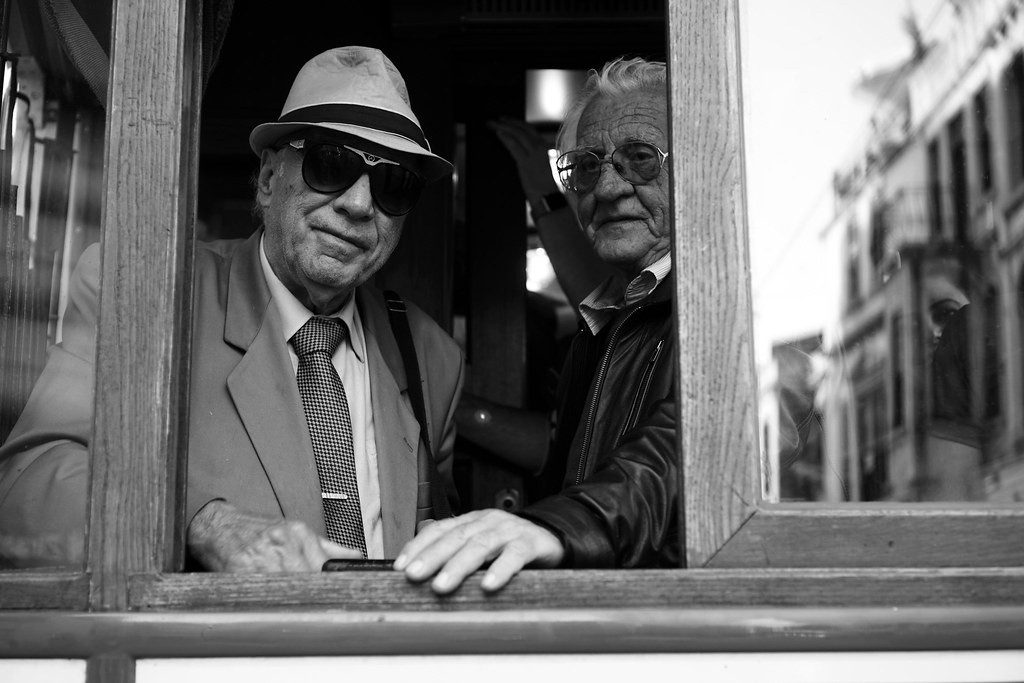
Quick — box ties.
[290,316,367,559]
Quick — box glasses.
[274,138,423,216]
[556,141,669,193]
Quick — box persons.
[0,47,468,574]
[393,55,680,595]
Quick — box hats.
[248,46,455,180]
